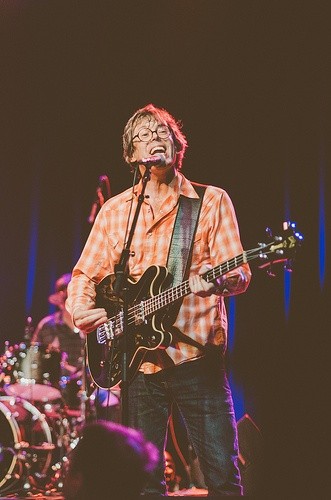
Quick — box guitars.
[0,396,52,497]
[86,220,303,392]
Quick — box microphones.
[130,152,167,169]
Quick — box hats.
[48,273,71,311]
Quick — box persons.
[64,104,253,499]
[23,272,121,407]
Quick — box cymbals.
[48,290,68,305]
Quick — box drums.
[2,342,68,400]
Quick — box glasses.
[131,125,171,142]
